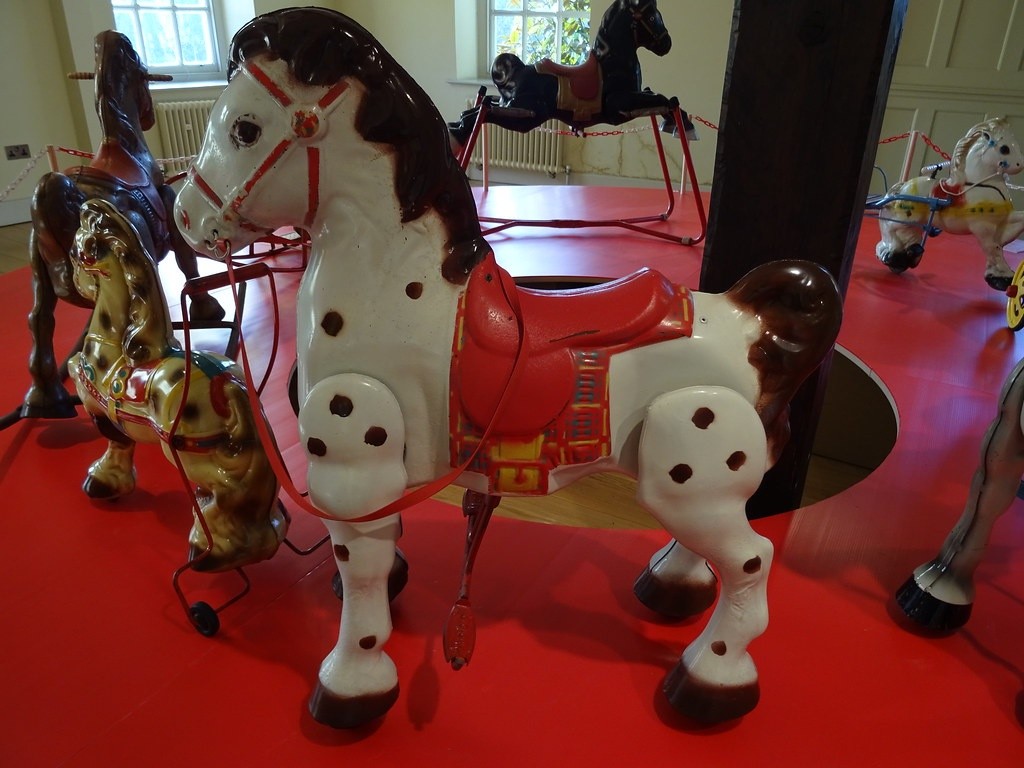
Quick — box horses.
[876,116,1024,632]
[22,0,842,730]
